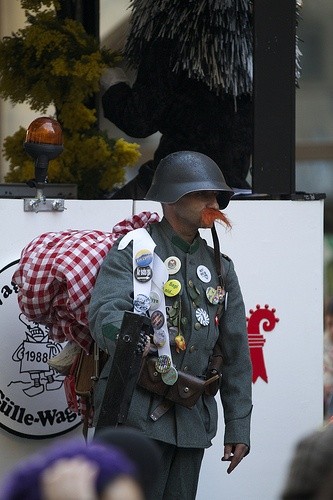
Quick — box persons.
[97,33,253,200]
[83,151,253,500]
[0,426,160,500]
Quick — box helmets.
[144,150,234,210]
